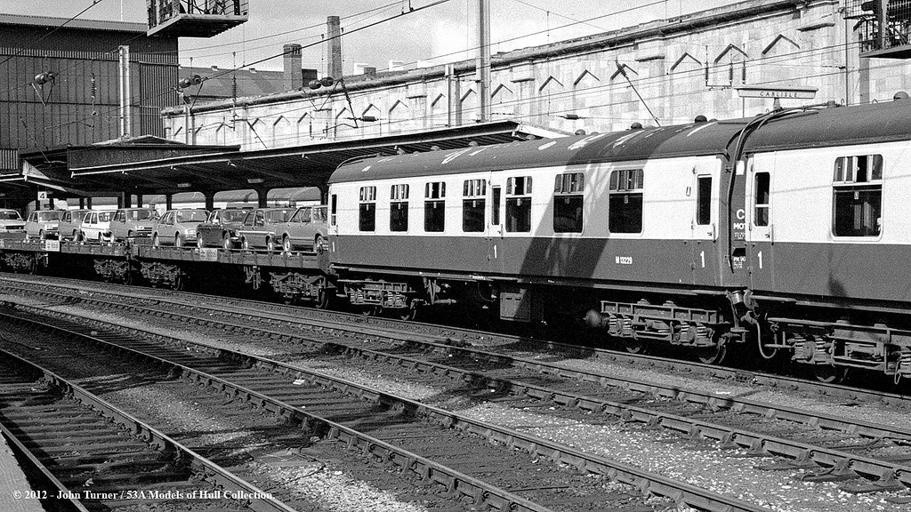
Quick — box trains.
[0,89,911,397]
